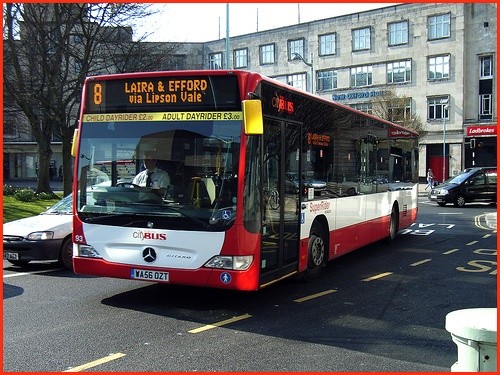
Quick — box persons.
[424,169,436,190]
[131,151,170,195]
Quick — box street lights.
[443,104,451,183]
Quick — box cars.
[3,180,146,275]
[285,172,327,194]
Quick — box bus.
[71,70,419,290]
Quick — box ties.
[145,172,154,187]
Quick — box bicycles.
[262,184,281,210]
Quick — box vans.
[429,167,497,207]
